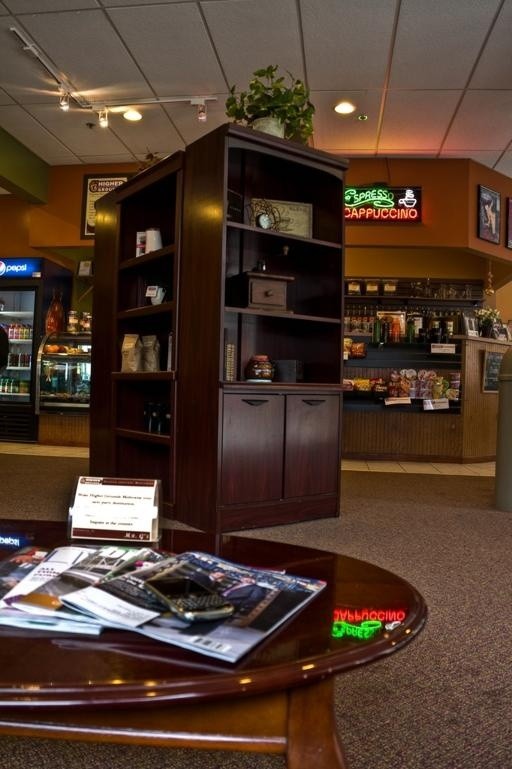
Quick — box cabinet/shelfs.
[37,332,90,407]
[346,277,484,413]
[86,120,350,538]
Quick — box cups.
[144,228,163,255]
[398,198,418,208]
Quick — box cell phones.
[145,575,239,621]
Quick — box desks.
[0,518,429,766]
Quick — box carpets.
[0,451,512,768]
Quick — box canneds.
[67,311,92,332]
[0,353,31,393]
[248,355,273,379]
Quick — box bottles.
[343,303,464,345]
[0,376,20,393]
[0,321,33,339]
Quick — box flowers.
[472,302,504,321]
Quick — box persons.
[1,324,10,375]
[480,192,500,241]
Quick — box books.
[227,342,234,383]
[223,328,229,384]
[0,537,330,662]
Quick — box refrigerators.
[0,255,75,444]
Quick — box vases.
[481,322,493,337]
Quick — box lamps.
[59,88,207,129]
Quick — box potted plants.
[225,60,316,145]
